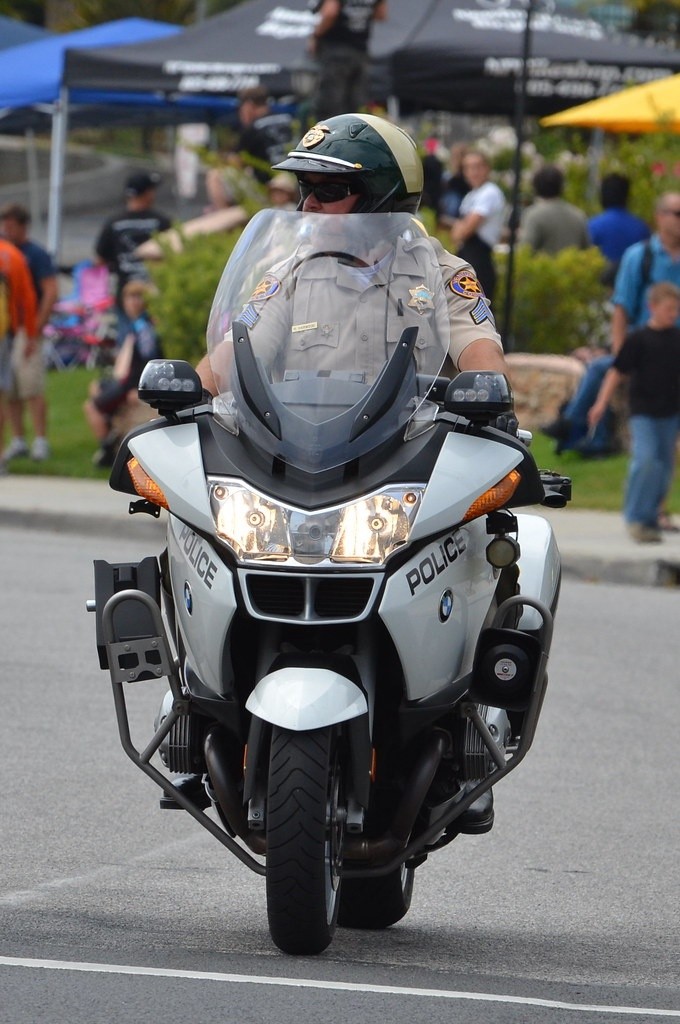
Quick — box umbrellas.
[539,73,679,137]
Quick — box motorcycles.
[84,207,564,954]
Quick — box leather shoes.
[160,776,210,809]
[463,779,495,833]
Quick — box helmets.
[271,114,424,215]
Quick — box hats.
[123,173,162,195]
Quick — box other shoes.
[91,429,122,464]
[4,438,29,460]
[31,436,51,459]
[539,417,575,440]
[629,523,660,543]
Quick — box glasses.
[298,179,373,204]
[663,208,680,217]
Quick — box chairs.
[51,261,118,370]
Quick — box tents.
[48,0,680,268]
[0,16,302,262]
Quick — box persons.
[0,235,38,458]
[451,149,510,314]
[586,283,680,540]
[542,339,617,459]
[158,114,520,834]
[95,171,171,308]
[83,280,165,472]
[588,176,648,287]
[207,85,298,206]
[418,139,471,219]
[306,1,388,119]
[609,191,680,356]
[586,171,651,261]
[0,203,58,463]
[515,165,593,256]
